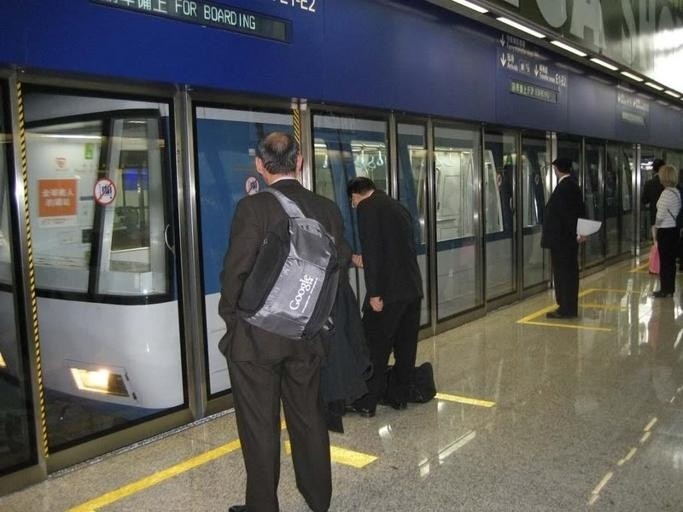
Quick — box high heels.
[653,288,675,297]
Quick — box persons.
[319,223,378,434]
[536,152,592,321]
[650,164,682,298]
[215,126,348,511]
[642,157,667,248]
[347,173,427,421]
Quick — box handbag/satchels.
[384,360,437,403]
[648,241,661,275]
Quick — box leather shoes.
[228,503,249,511]
[345,402,375,418]
[546,309,578,319]
[378,397,408,410]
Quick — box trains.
[1,82,682,427]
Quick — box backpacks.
[237,188,345,344]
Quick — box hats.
[551,157,573,170]
